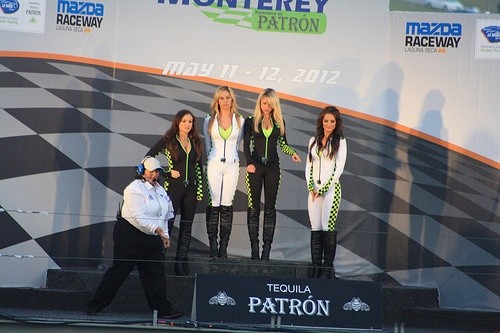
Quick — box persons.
[85,156,185,317]
[204,85,245,261]
[144,109,205,275]
[305,106,347,281]
[243,88,301,261]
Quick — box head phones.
[137,157,150,176]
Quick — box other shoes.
[161,311,186,319]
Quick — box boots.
[206,205,221,260]
[322,231,337,279]
[261,209,276,260]
[247,207,260,260]
[174,225,192,277]
[219,206,234,258]
[310,230,323,279]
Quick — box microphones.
[153,178,156,182]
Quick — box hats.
[142,156,164,171]
